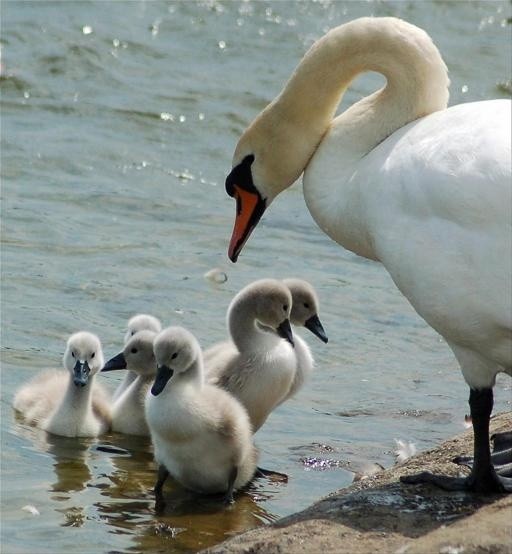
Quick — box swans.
[222,11,512,499]
[11,273,330,506]
